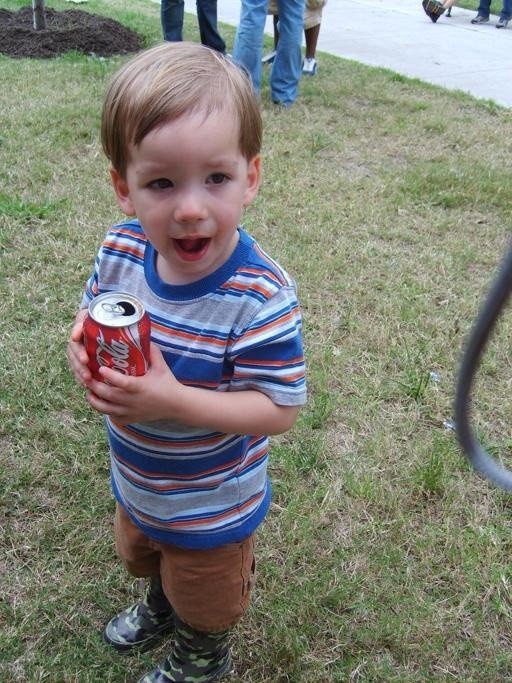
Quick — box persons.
[257,0,329,76]
[232,0,306,110]
[162,0,234,61]
[421,0,459,24]
[472,0,511,29]
[67,40,312,681]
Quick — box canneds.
[84,291,151,396]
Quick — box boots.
[136,617,231,683]
[105,562,177,654]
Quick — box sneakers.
[423,0,509,28]
[301,56,317,75]
[262,49,276,64]
